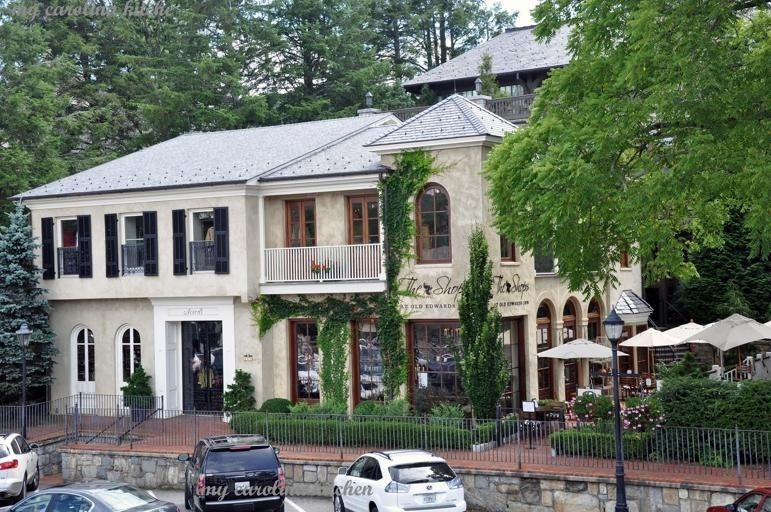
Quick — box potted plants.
[119,364,153,421]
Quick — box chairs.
[620,375,656,400]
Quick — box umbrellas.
[538,312,771,397]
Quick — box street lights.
[603,308,630,512]
[15,323,33,438]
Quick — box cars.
[1,480,180,512]
[0,432,40,501]
[706,488,770,512]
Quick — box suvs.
[178,434,286,512]
[332,450,467,512]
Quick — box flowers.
[310,261,331,274]
